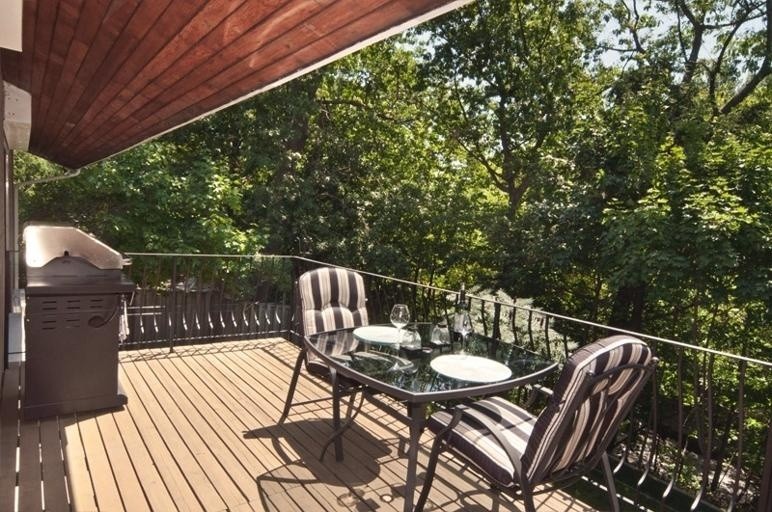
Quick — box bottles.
[430,323,451,345]
[454,290,468,342]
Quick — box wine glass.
[454,312,472,354]
[390,304,410,350]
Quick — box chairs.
[415,334,660,512]
[278,268,370,461]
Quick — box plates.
[430,354,513,383]
[352,326,413,344]
